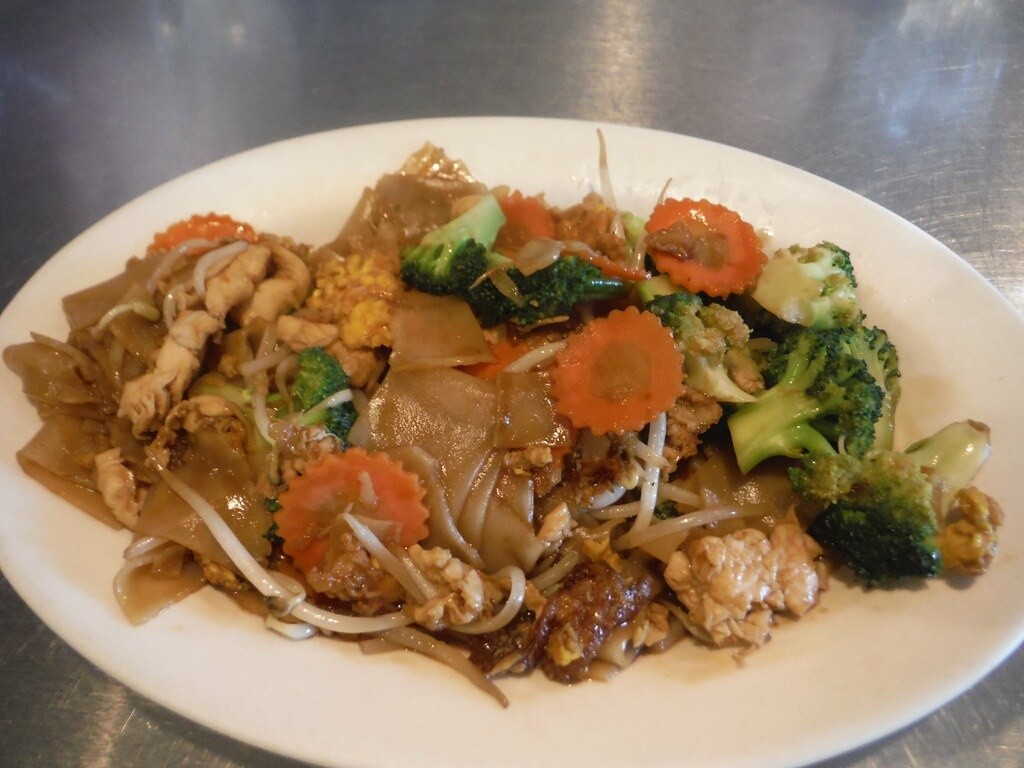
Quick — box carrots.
[148,189,769,579]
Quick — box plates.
[0,115,1024,768]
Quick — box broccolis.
[213,197,991,592]
